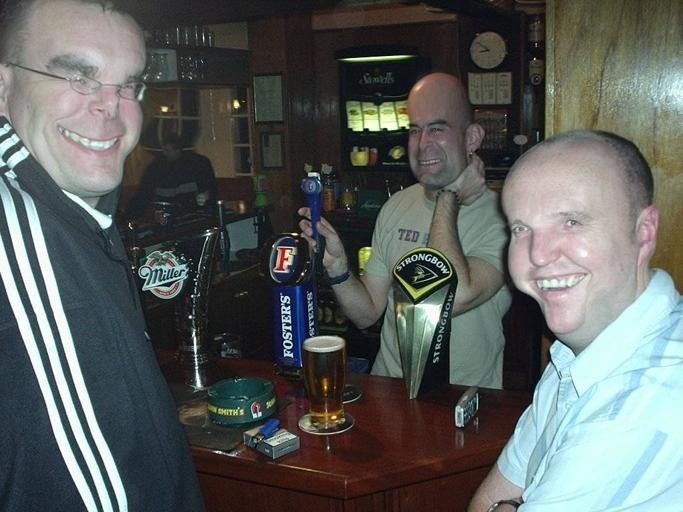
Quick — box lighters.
[250,416,281,444]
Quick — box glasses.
[4,56,148,106]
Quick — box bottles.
[321,165,340,213]
[213,200,230,278]
[127,218,145,294]
[526,16,545,87]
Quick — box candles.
[470,31,508,70]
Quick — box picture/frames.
[249,70,287,124]
[261,131,286,170]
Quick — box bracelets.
[434,188,462,216]
[486,499,520,512]
[324,268,352,289]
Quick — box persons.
[291,67,525,397]
[464,122,682,512]
[0,1,210,512]
[129,129,216,210]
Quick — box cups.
[210,333,243,360]
[350,147,379,166]
[341,192,358,210]
[303,336,347,429]
[138,17,216,84]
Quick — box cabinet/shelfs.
[338,53,431,174]
[142,41,249,90]
[455,11,527,181]
